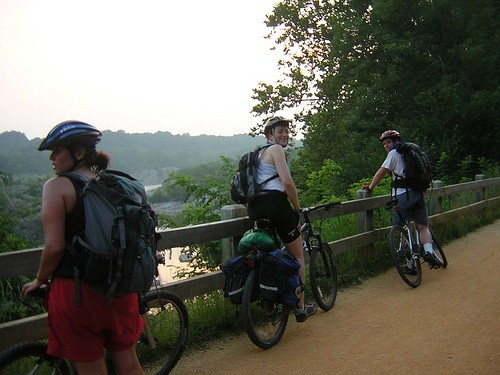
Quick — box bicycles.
[1,250,189,375]
[385,197,448,289]
[240,201,343,350]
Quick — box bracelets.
[36,277,48,283]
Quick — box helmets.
[379,129,401,141]
[37,121,102,151]
[264,115,292,135]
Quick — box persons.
[362,129,443,269]
[22,121,146,375]
[247,116,317,322]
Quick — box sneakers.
[423,250,441,266]
[294,302,318,322]
[264,300,275,315]
[406,257,416,269]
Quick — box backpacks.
[219,254,260,304]
[389,142,430,202]
[59,168,161,305]
[257,248,305,309]
[230,143,280,206]
